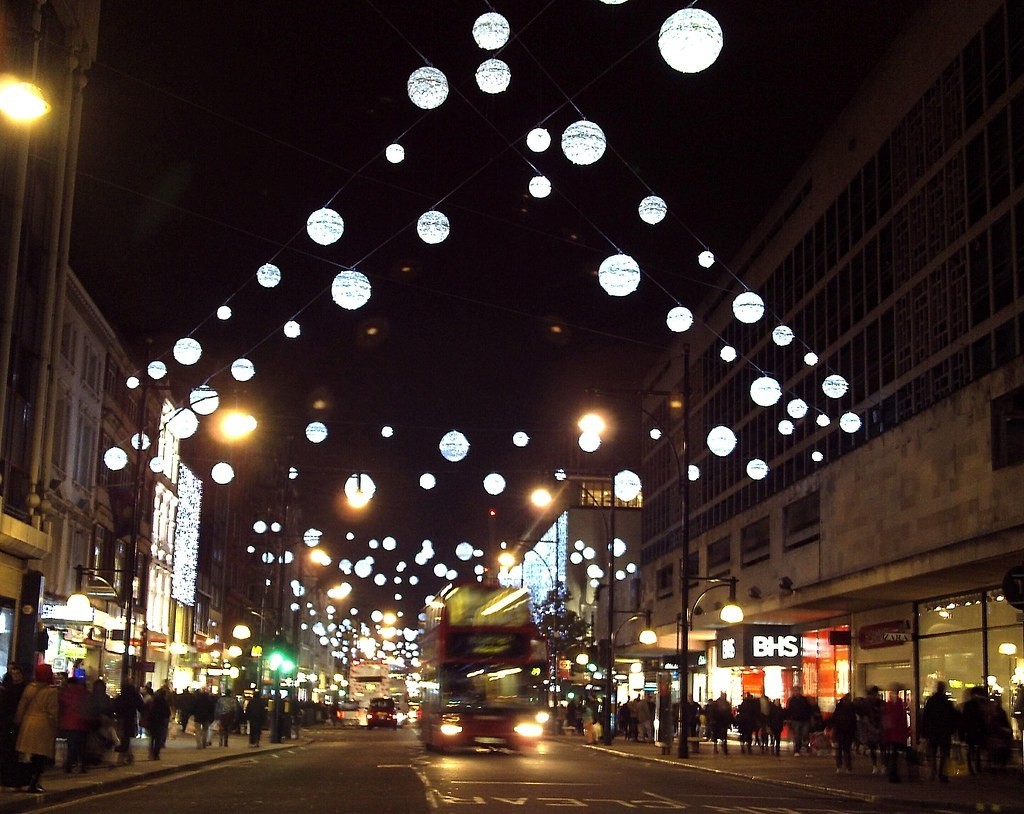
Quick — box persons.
[554,678,1024,793]
[2,654,337,799]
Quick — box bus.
[415,581,550,754]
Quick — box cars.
[335,697,424,729]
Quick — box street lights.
[68,376,260,694]
[230,468,374,695]
[570,385,748,761]
[494,533,593,738]
[530,467,660,747]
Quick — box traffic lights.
[269,641,296,677]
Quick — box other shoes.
[586,742,598,745]
[181,729,186,732]
[114,745,128,752]
[714,741,837,757]
[579,732,584,735]
[70,762,84,772]
[890,775,899,782]
[177,721,183,724]
[931,768,936,778]
[706,735,712,741]
[971,772,981,779]
[939,773,948,779]
[28,784,45,793]
[199,744,206,749]
[871,766,886,775]
[615,728,656,743]
[206,742,212,746]
[249,744,260,748]
[834,768,855,774]
[220,743,228,747]
[153,751,160,760]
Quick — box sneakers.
[136,733,149,739]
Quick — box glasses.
[11,672,21,675]
